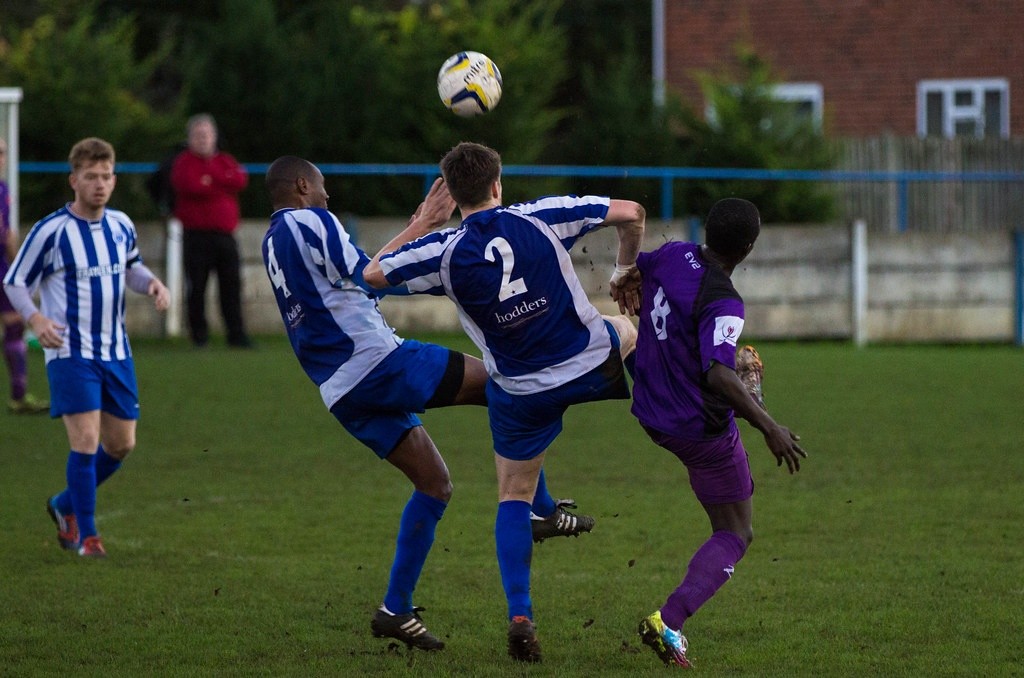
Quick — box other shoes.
[6,395,51,415]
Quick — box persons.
[363,143,645,663]
[260,158,595,650]
[630,198,807,669]
[3,138,171,555]
[0,139,51,414]
[169,113,252,351]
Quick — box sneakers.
[78,536,105,555]
[47,494,80,549]
[530,500,594,543]
[736,343,765,409]
[639,610,695,668]
[370,603,445,651]
[507,616,542,663]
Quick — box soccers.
[436,50,504,120]
